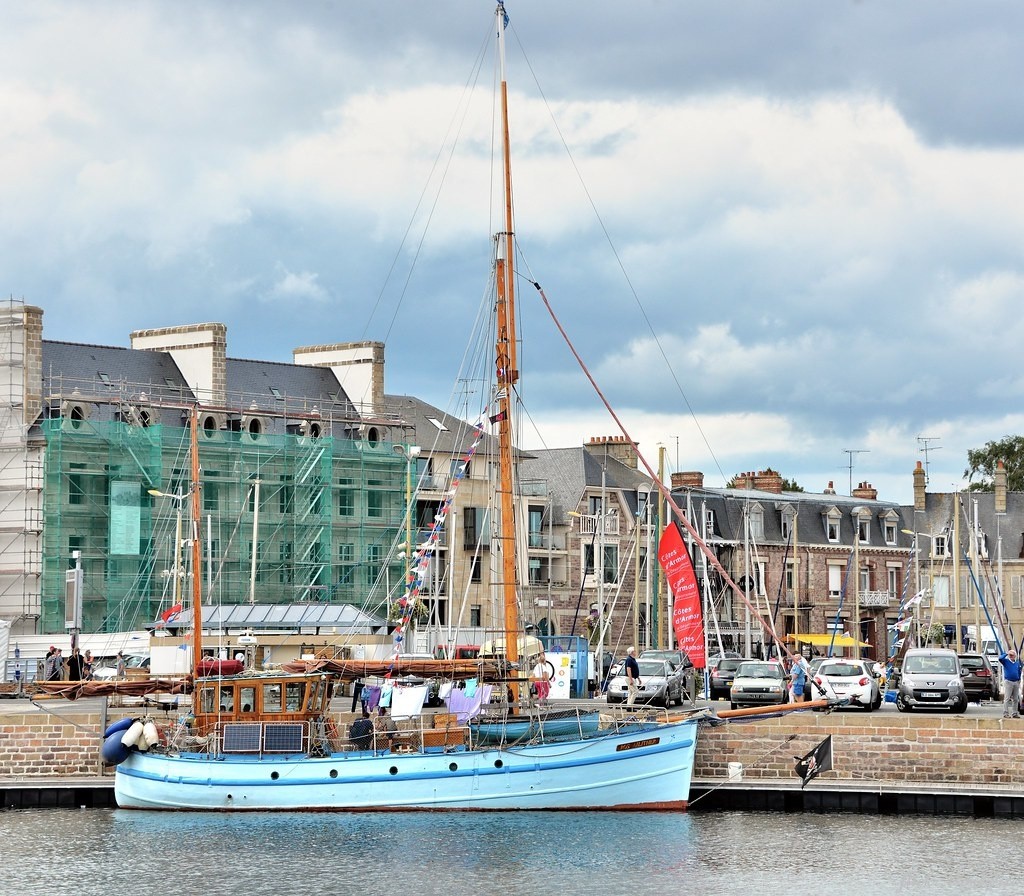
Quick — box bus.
[477,637,545,703]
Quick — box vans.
[388,654,435,661]
[943,625,969,651]
[967,626,998,673]
[893,648,970,714]
[434,645,481,659]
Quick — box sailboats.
[104,1,849,813]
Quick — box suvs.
[956,654,1000,700]
[572,651,619,687]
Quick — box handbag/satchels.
[530,684,538,695]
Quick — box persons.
[243,704,250,712]
[220,705,226,713]
[46,646,93,682]
[117,653,124,682]
[625,646,641,712]
[998,650,1021,719]
[350,713,376,750]
[756,644,889,714]
[380,712,402,751]
[229,706,233,713]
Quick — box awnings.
[784,633,872,647]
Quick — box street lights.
[901,530,921,648]
[145,486,203,612]
[401,447,423,654]
[568,508,616,683]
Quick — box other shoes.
[1004,715,1011,718]
[1012,713,1020,718]
[626,707,638,712]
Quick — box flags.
[161,601,182,624]
[497,0,509,39]
[887,589,924,648]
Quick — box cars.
[805,658,882,712]
[730,660,792,710]
[93,653,150,682]
[607,658,686,708]
[609,659,628,676]
[637,648,695,697]
